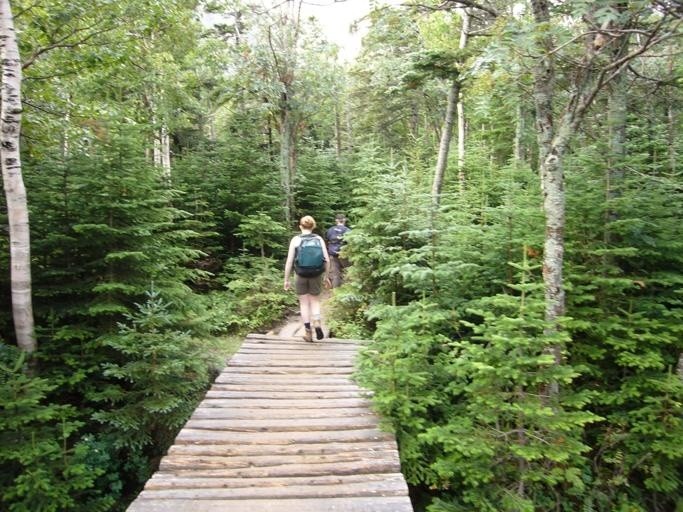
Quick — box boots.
[302,328,312,342]
[313,318,324,340]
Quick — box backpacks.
[293,234,326,278]
[328,226,349,257]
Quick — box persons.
[324,213,352,290]
[284,214,330,342]
